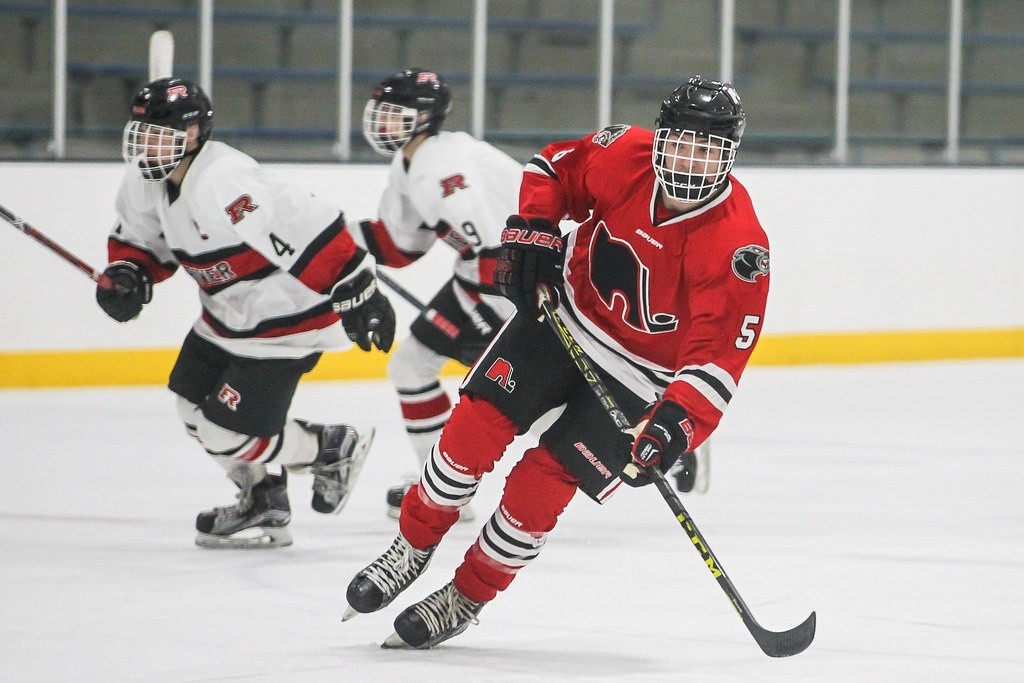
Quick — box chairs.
[0,0,1024,167]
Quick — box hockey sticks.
[377,269,463,342]
[535,285,818,660]
[0,202,135,300]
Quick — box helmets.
[650,75,748,204]
[362,67,453,158]
[121,77,212,184]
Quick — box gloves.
[493,215,565,317]
[620,399,696,487]
[97,259,150,322]
[331,268,396,354]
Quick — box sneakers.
[380,579,485,649]
[341,528,444,623]
[386,478,475,522]
[289,417,376,516]
[194,463,294,549]
[668,438,710,500]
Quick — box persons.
[97,77,395,549]
[356,68,712,524]
[341,75,771,649]
